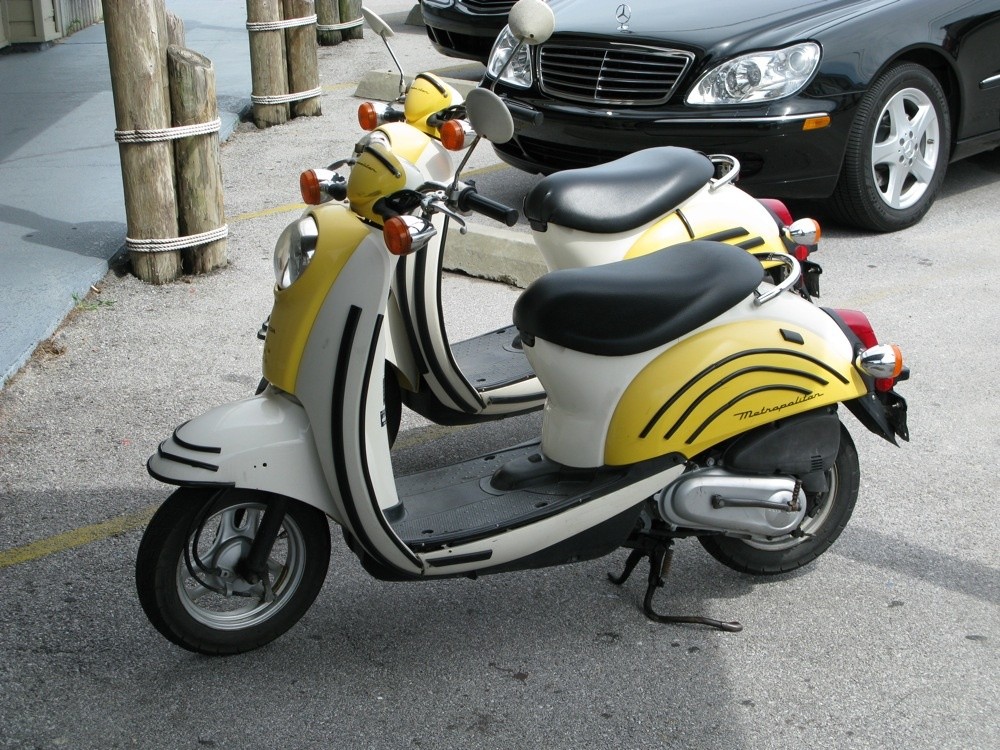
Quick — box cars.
[413,0,1000,236]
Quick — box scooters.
[132,77,911,661]
[259,4,827,459]
